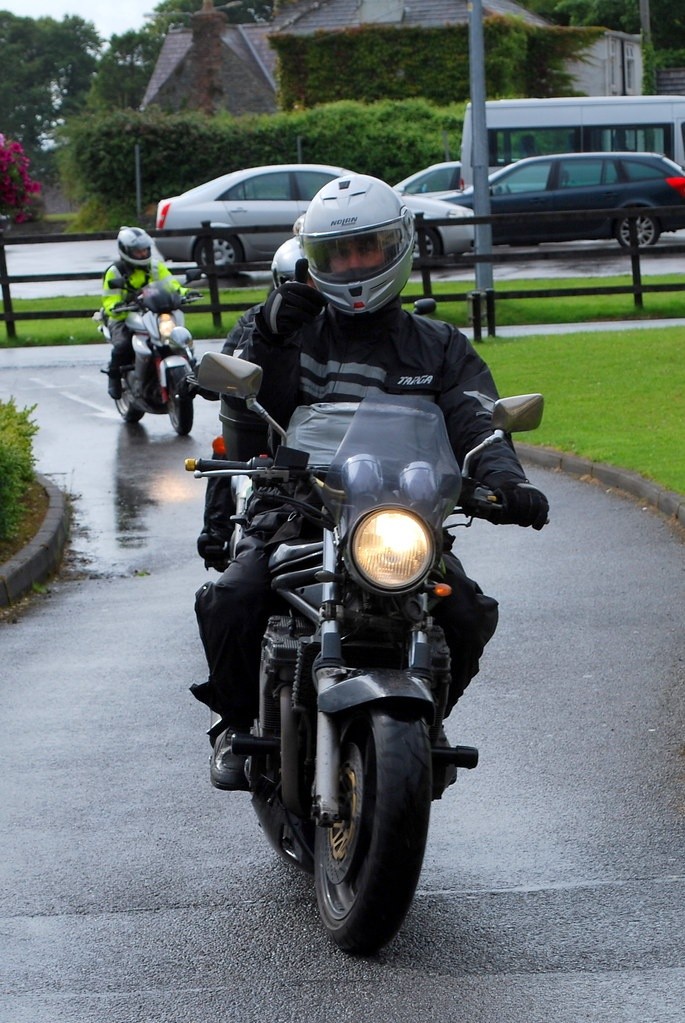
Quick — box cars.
[386,162,460,197]
[150,163,476,280]
[435,151,685,248]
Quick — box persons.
[176,237,315,401]
[186,175,550,792]
[102,225,202,400]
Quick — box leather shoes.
[106,365,122,401]
[210,720,248,792]
[431,727,458,787]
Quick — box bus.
[459,93,685,188]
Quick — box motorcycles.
[92,268,205,438]
[183,351,545,958]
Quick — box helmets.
[297,173,416,317]
[116,225,152,267]
[269,235,308,289]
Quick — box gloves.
[184,288,204,305]
[262,258,328,339]
[480,479,549,533]
